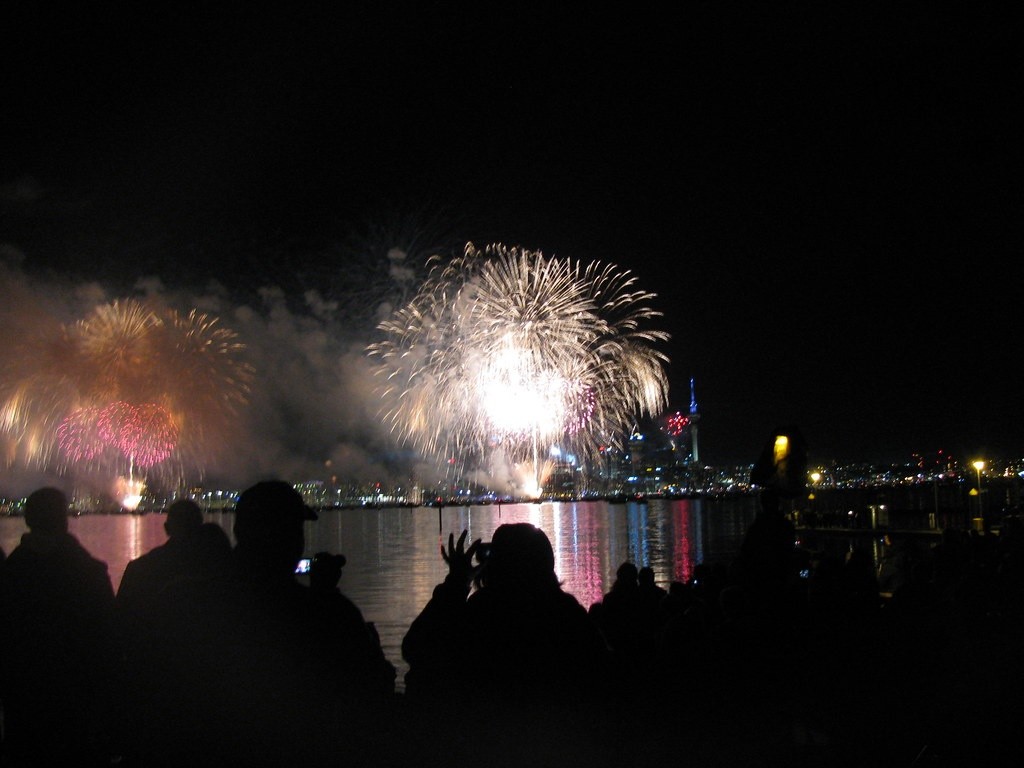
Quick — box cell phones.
[690,578,701,586]
[294,558,313,575]
[796,567,814,582]
[475,542,493,563]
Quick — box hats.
[235,482,318,522]
